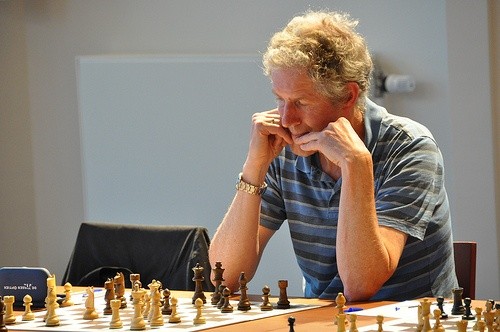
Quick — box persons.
[208,11,460,302]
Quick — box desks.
[2,286,500,332]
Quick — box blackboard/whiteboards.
[72,54,377,299]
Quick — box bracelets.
[235,172,268,195]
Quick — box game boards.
[0,265,324,332]
[333,272,500,332]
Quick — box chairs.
[61,222,215,292]
[453,242,477,299]
